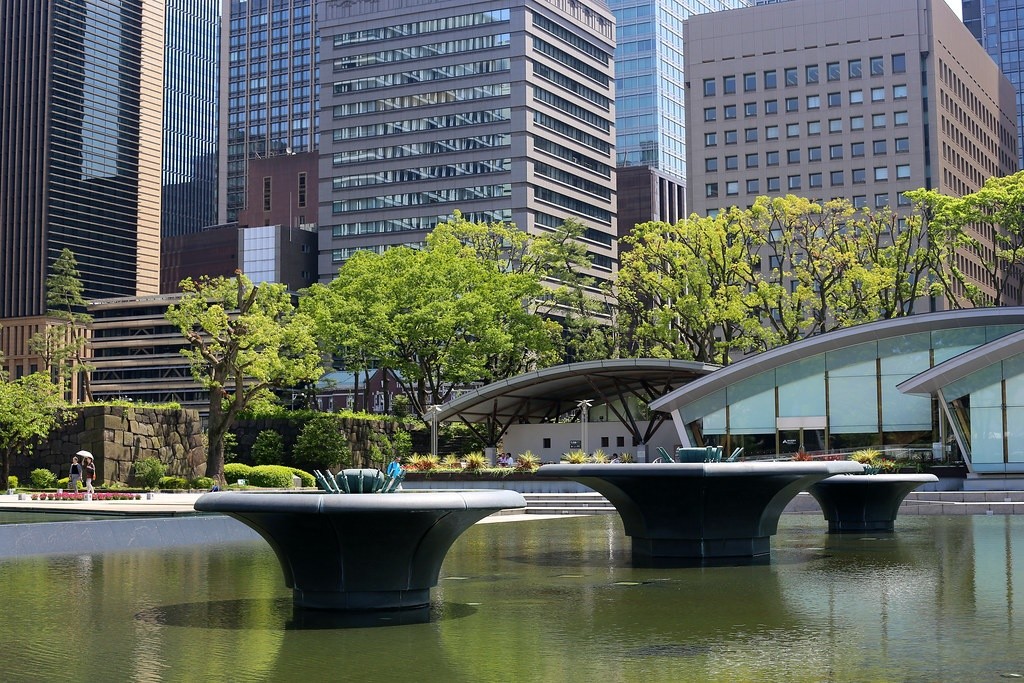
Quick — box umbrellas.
[76,450,94,461]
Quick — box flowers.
[31,493,141,500]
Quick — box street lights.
[428,405,443,455]
[575,399,594,456]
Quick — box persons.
[64,396,155,405]
[83,457,95,494]
[589,453,620,465]
[387,456,408,490]
[496,451,521,468]
[69,457,82,494]
